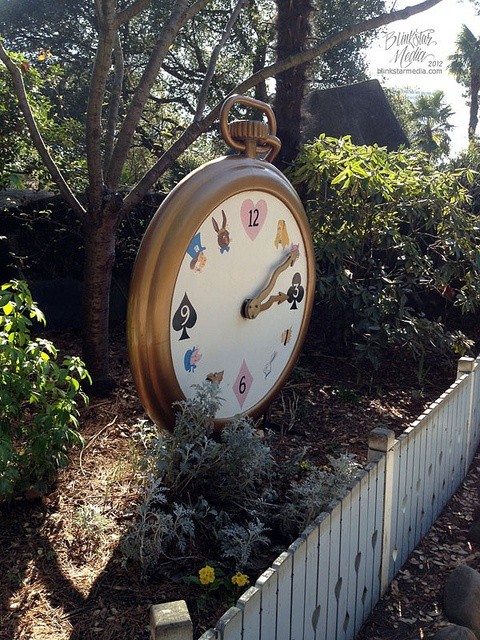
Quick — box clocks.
[129,96,318,445]
